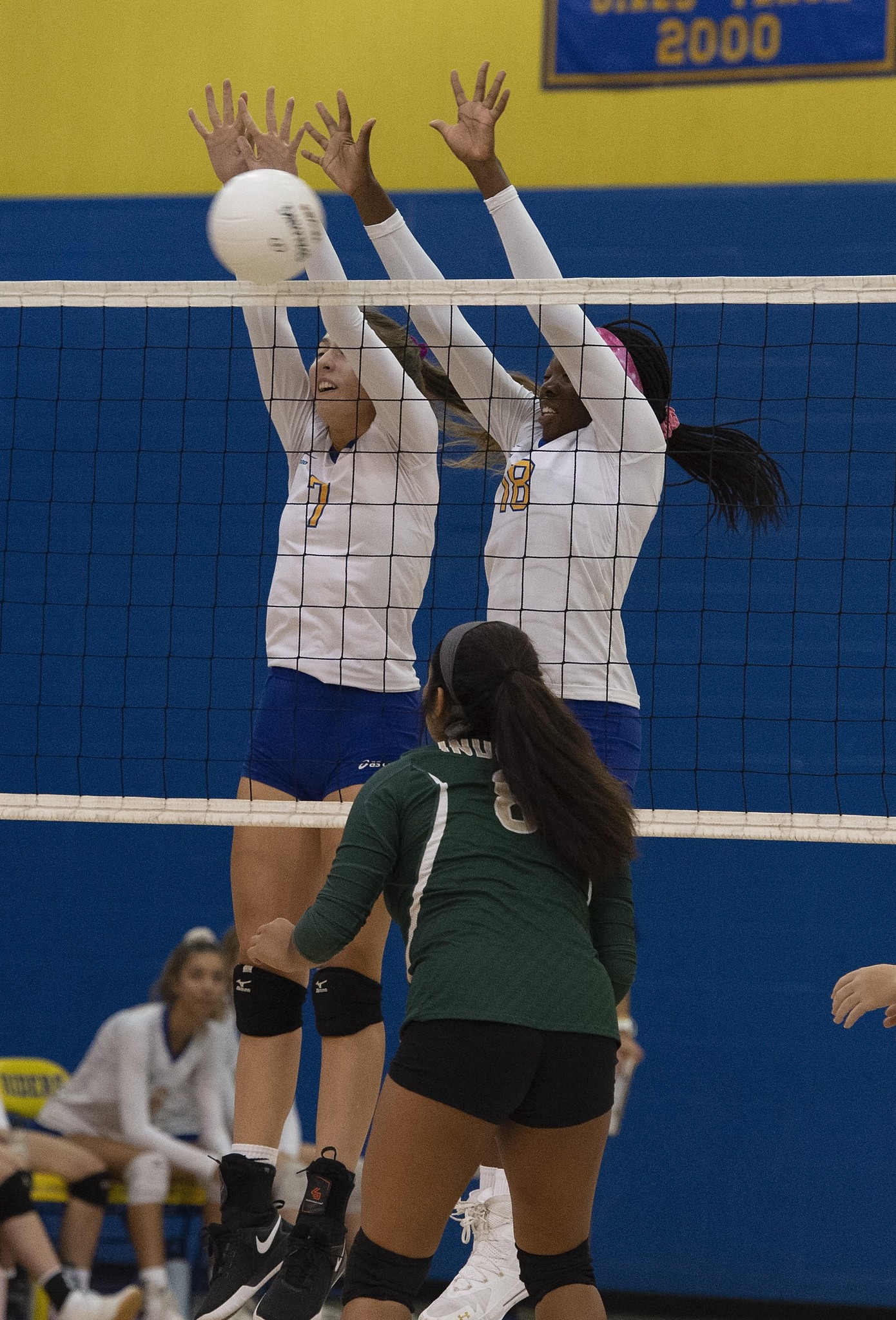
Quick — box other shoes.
[141,1279,185,1320]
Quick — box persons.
[615,994,646,1077]
[246,619,638,1320]
[299,60,793,1320]
[0,924,367,1320]
[183,79,539,1320]
[831,963,896,1029]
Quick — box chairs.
[0,1056,208,1257]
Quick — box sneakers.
[48,1283,141,1320]
[193,1153,297,1320]
[249,1145,355,1320]
[414,1186,530,1320]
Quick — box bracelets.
[617,1017,637,1035]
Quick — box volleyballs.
[203,168,329,286]
